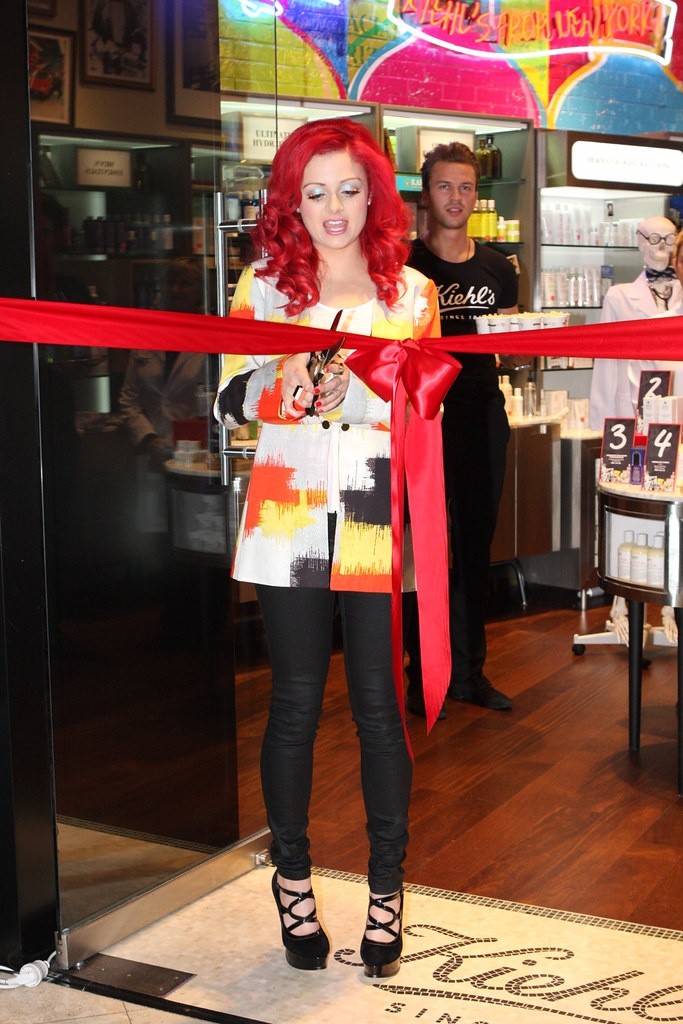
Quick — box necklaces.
[432,235,474,262]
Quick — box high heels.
[360,889,404,978]
[271,870,330,970]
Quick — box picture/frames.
[27,1,221,130]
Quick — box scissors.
[306,308,346,417]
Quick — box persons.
[406,141,530,708]
[214,118,441,977]
[628,231,683,418]
[116,260,214,547]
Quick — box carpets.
[96,856,683,1024]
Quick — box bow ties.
[646,265,677,282]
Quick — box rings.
[337,362,346,375]
[292,386,307,405]
[332,387,341,396]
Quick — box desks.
[508,417,683,790]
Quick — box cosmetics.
[467,198,683,587]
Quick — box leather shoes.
[406,689,446,719]
[447,675,514,708]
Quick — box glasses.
[636,230,677,245]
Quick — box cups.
[476,313,570,334]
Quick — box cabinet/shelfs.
[222,86,683,674]
[35,179,210,269]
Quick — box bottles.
[539,266,602,307]
[486,133,502,179]
[225,190,261,221]
[498,374,537,421]
[474,139,491,178]
[465,199,520,243]
[82,213,174,251]
[540,203,600,246]
